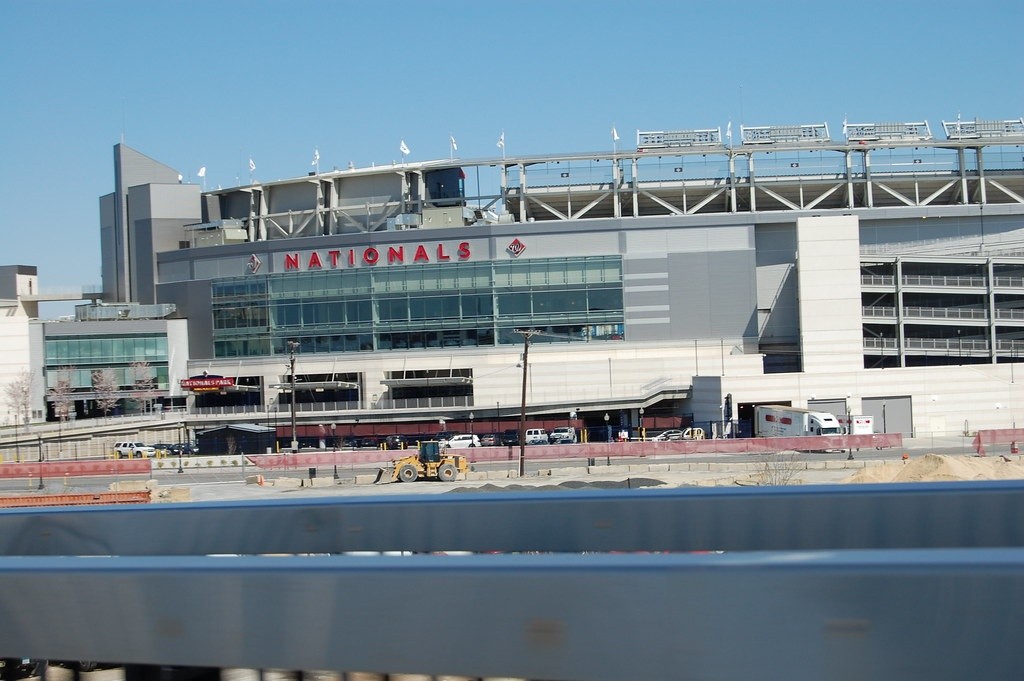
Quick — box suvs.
[114,442,156,457]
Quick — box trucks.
[835,414,874,436]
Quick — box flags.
[451,136,457,151]
[726,122,731,139]
[611,128,619,140]
[400,141,410,155]
[197,168,205,176]
[312,153,319,165]
[250,159,256,171]
[496,132,504,147]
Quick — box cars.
[438,434,482,448]
[481,434,500,447]
[549,426,574,443]
[500,432,520,445]
[361,437,379,446]
[147,442,199,456]
[382,435,408,449]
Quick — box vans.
[524,428,549,445]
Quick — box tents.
[196,424,276,455]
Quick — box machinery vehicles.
[373,439,469,485]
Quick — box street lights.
[469,411,477,449]
[637,407,644,443]
[604,412,612,466]
[176,421,184,474]
[37,431,43,489]
[330,422,339,480]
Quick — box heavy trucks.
[754,404,848,454]
